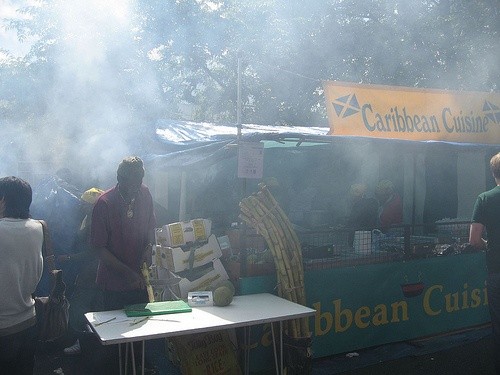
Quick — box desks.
[83,292,317,375]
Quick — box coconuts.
[206,279,235,306]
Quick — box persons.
[343,178,402,244]
[0,157,158,375]
[469,151,500,375]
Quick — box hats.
[82,187,106,204]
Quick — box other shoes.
[136,365,159,375]
[64,339,81,355]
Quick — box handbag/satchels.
[33,292,71,343]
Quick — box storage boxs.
[154,218,265,375]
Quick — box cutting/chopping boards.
[123,301,192,316]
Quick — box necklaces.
[117,184,136,217]
